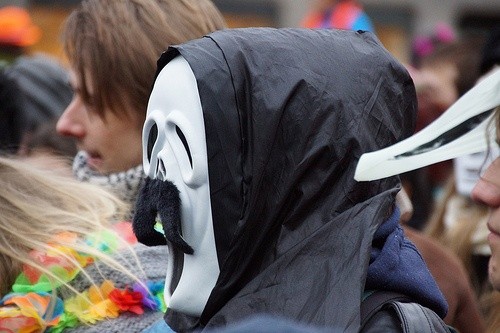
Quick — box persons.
[300,1,500,333]
[55,1,228,333]
[139,26,467,333]
[411,36,467,129]
[0,6,83,162]
[0,149,169,333]
